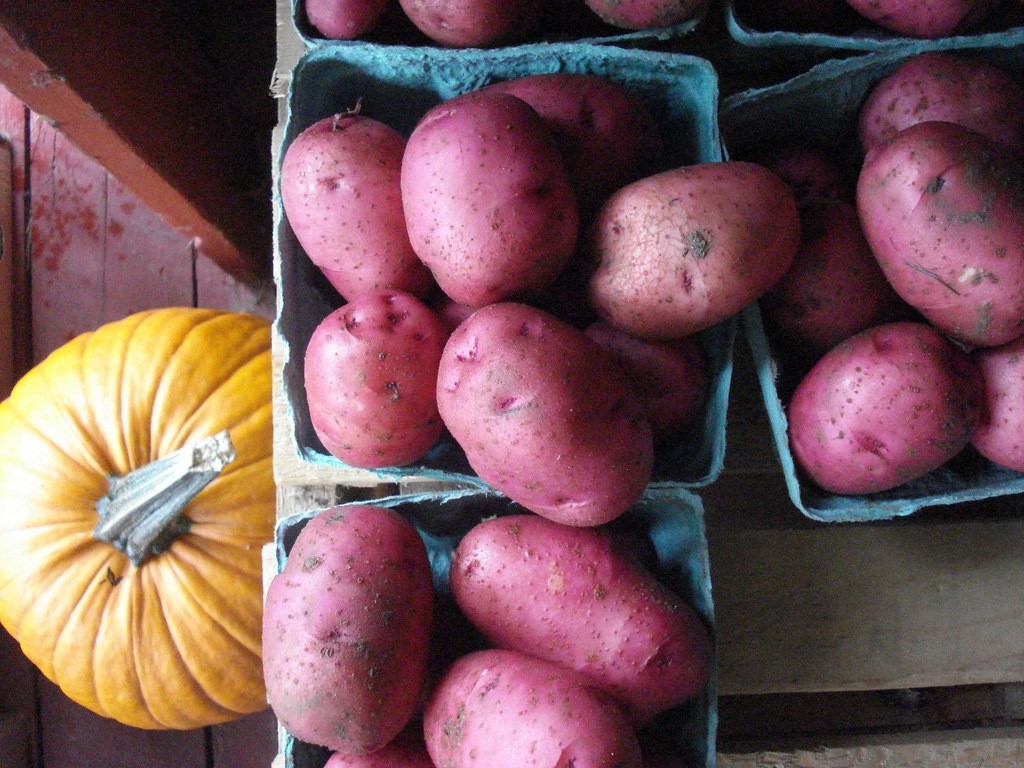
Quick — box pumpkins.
[0,306,280,729]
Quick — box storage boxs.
[256,0,1024,768]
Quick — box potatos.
[262,0,1024,768]
